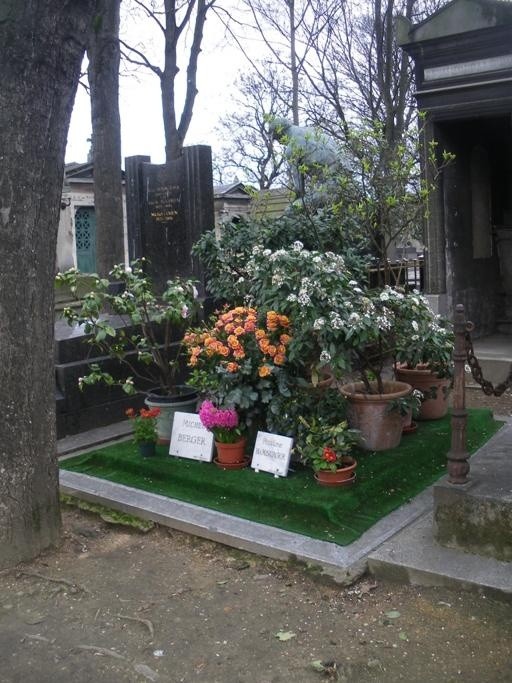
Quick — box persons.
[266,117,353,214]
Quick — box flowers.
[199,398,243,443]
[56,238,453,394]
[300,420,362,470]
[128,407,160,440]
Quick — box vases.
[402,397,418,433]
[314,456,357,486]
[138,440,156,457]
[213,438,251,469]
[143,385,198,445]
[339,380,414,450]
[391,361,456,420]
